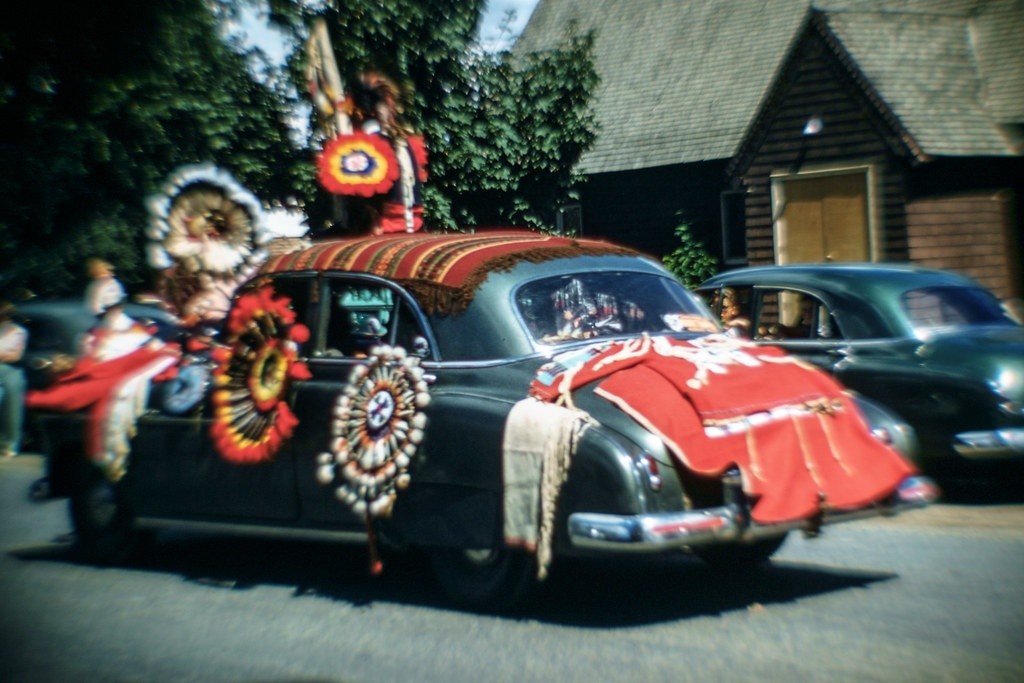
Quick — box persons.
[0,297,28,461]
[712,292,753,340]
[84,251,131,310]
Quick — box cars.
[39,228,947,609]
[7,302,188,451]
[690,261,1023,498]
